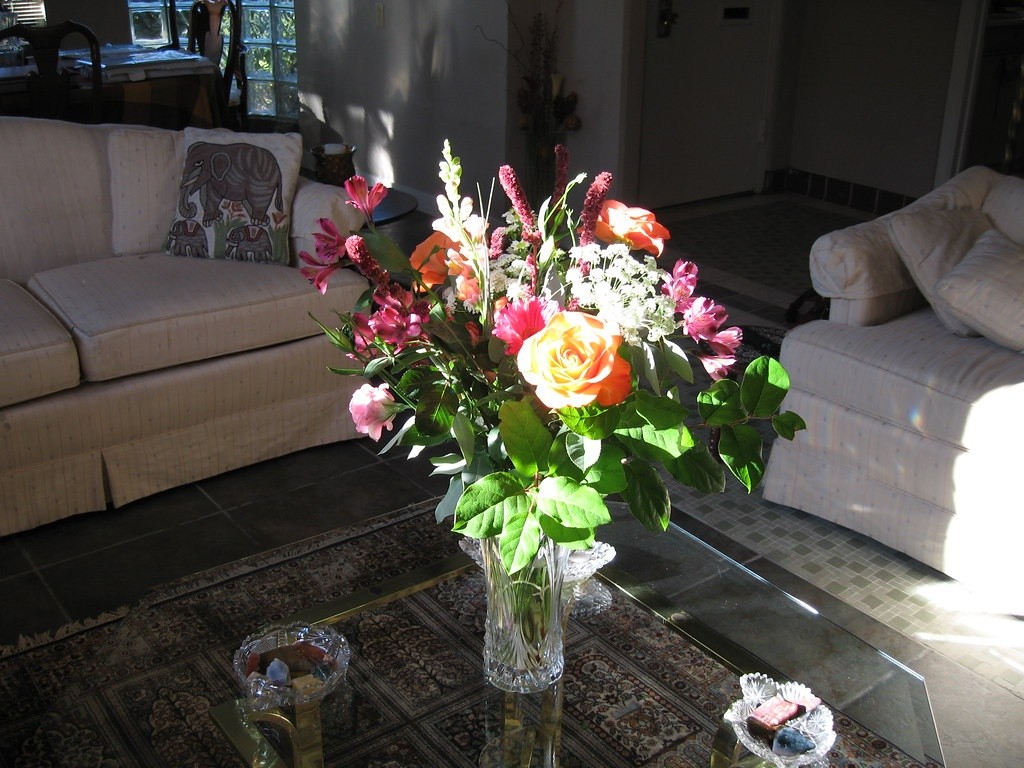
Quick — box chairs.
[188,0,237,128]
[0,18,102,125]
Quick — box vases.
[309,143,358,190]
[524,105,560,213]
[480,533,565,692]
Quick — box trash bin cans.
[309,143,357,185]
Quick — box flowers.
[473,0,583,133]
[287,127,806,577]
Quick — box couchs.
[0,114,372,534]
[761,164,1023,616]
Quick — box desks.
[0,44,221,131]
[363,188,418,228]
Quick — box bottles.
[308,144,358,188]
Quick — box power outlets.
[376,2,384,29]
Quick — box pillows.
[930,230,1024,356]
[889,210,992,339]
[163,126,304,267]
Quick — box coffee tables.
[8,496,947,768]
